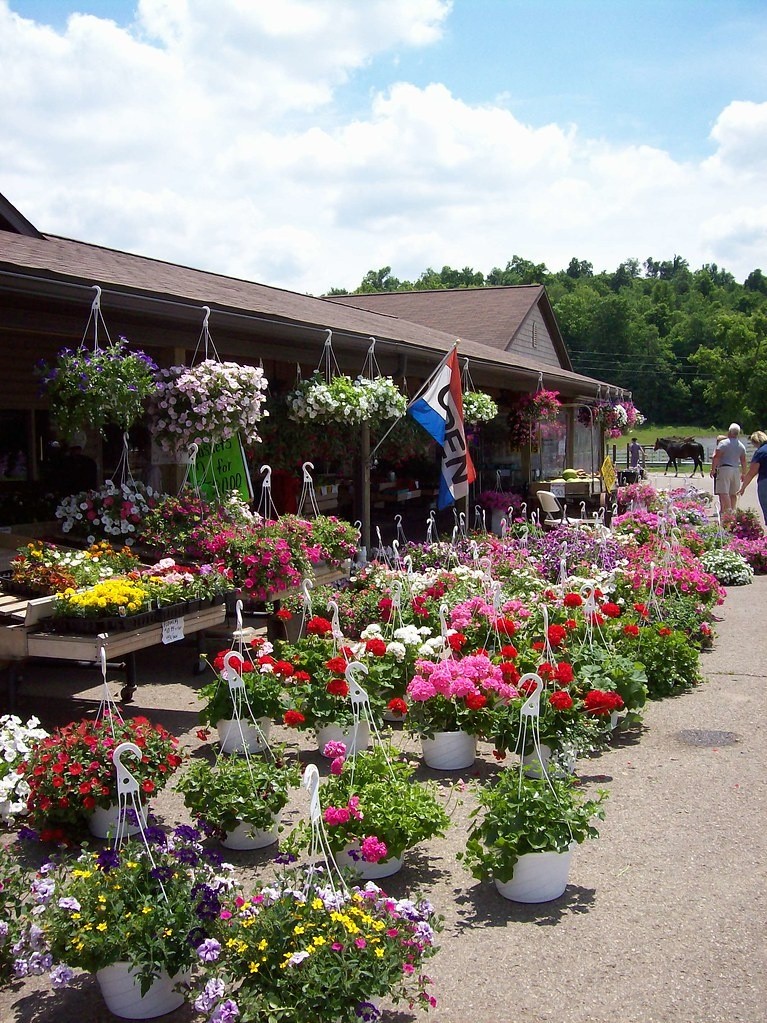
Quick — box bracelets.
[710,469,713,470]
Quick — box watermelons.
[552,469,579,482]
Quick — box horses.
[654,436,706,478]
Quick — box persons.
[737,431,767,525]
[57,446,97,481]
[629,438,647,467]
[712,434,727,495]
[710,424,746,512]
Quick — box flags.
[407,347,475,511]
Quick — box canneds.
[389,471,395,481]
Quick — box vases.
[420,732,475,769]
[314,721,368,758]
[216,716,270,754]
[218,810,278,850]
[88,802,148,838]
[521,744,577,779]
[96,960,191,1019]
[336,836,403,879]
[495,844,569,903]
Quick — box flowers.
[12,480,361,614]
[457,715,614,883]
[506,389,646,451]
[198,484,766,741]
[48,335,500,477]
[2,707,465,864]
[189,851,444,1023]
[0,809,235,999]
